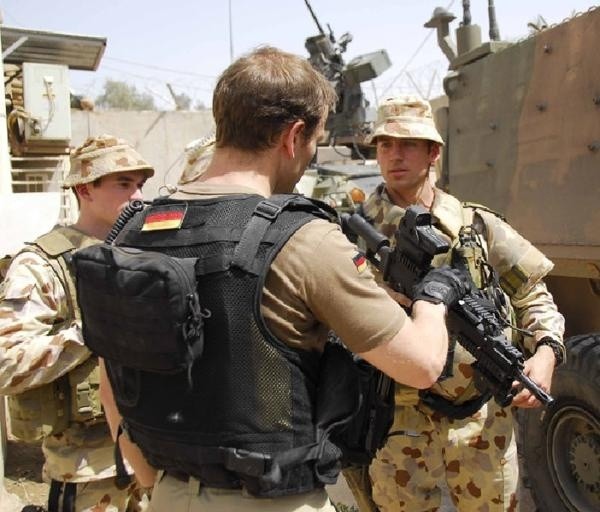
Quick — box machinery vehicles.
[297,0,600,512]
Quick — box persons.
[1,133,156,510]
[97,43,471,511]
[346,94,571,512]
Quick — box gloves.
[410,264,472,315]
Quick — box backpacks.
[71,243,212,408]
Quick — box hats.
[362,96,446,147]
[60,133,154,188]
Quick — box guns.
[341,204,554,407]
